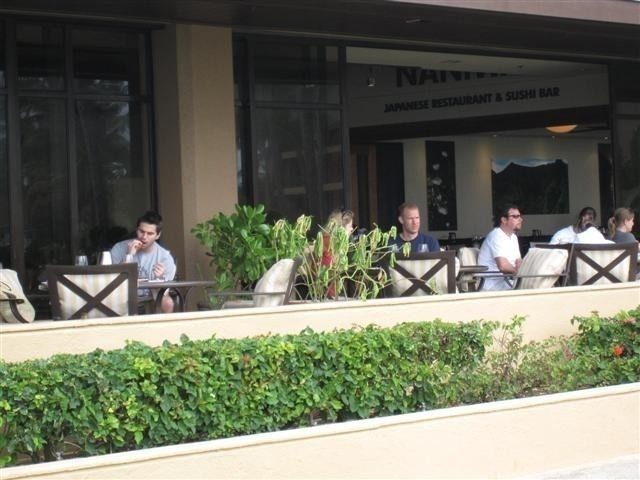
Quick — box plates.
[138,278,149,282]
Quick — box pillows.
[253,259,295,308]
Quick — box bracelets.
[123,253,133,259]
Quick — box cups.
[417,243,429,253]
[74,255,91,265]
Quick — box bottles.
[97,251,113,266]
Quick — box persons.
[111,210,177,315]
[604,205,640,282]
[377,201,442,256]
[472,204,523,292]
[310,208,386,300]
[547,207,614,247]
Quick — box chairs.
[0,261,140,324]
[205,253,304,309]
[381,242,639,296]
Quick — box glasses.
[508,214,521,219]
